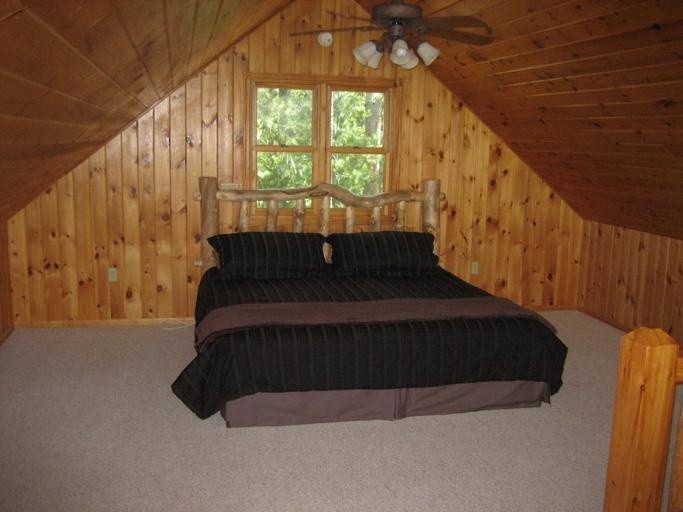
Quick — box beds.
[170,174,568,432]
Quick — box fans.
[287,1,496,46]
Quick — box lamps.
[350,23,440,70]
